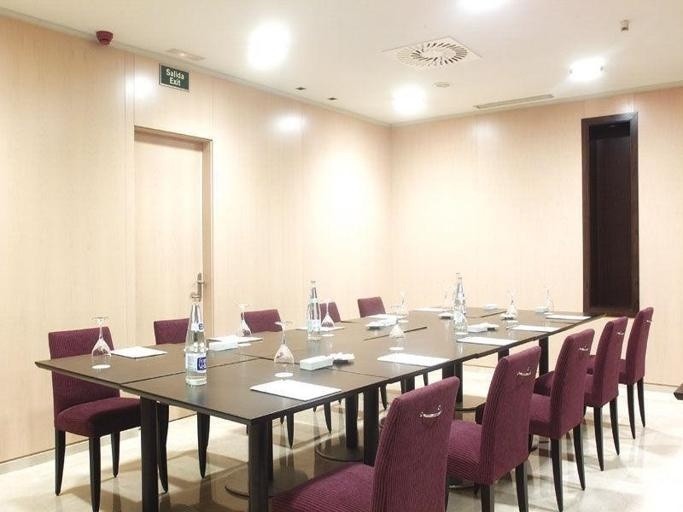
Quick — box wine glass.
[273,321,296,380]
[503,292,520,327]
[387,310,408,354]
[449,304,475,345]
[90,316,114,372]
[542,285,554,315]
[235,302,253,339]
[322,295,335,328]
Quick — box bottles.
[453,274,469,320]
[183,293,210,389]
[305,278,323,344]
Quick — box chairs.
[44,325,170,512]
[240,309,333,448]
[532,316,630,471]
[380,347,540,512]
[305,303,344,412]
[357,298,430,409]
[276,374,460,512]
[150,317,212,478]
[589,306,653,439]
[474,328,594,511]
